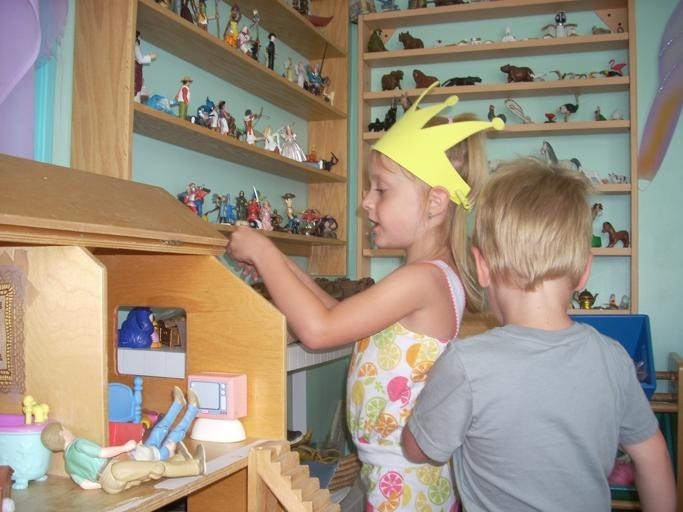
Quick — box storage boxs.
[564,313,657,401]
[609,414,675,501]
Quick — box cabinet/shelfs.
[0,155,342,512]
[610,351,682,512]
[356,0,638,341]
[72,0,353,281]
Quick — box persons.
[144,0,334,106]
[134,31,338,172]
[228,111,492,512]
[177,180,338,240]
[39,386,209,495]
[399,152,683,512]
